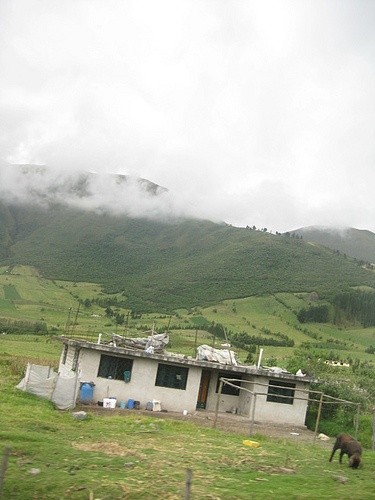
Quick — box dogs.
[329,432,362,470]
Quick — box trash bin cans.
[79,381,95,405]
[127,399,134,410]
[146,401,153,411]
[152,400,162,411]
[133,401,140,410]
